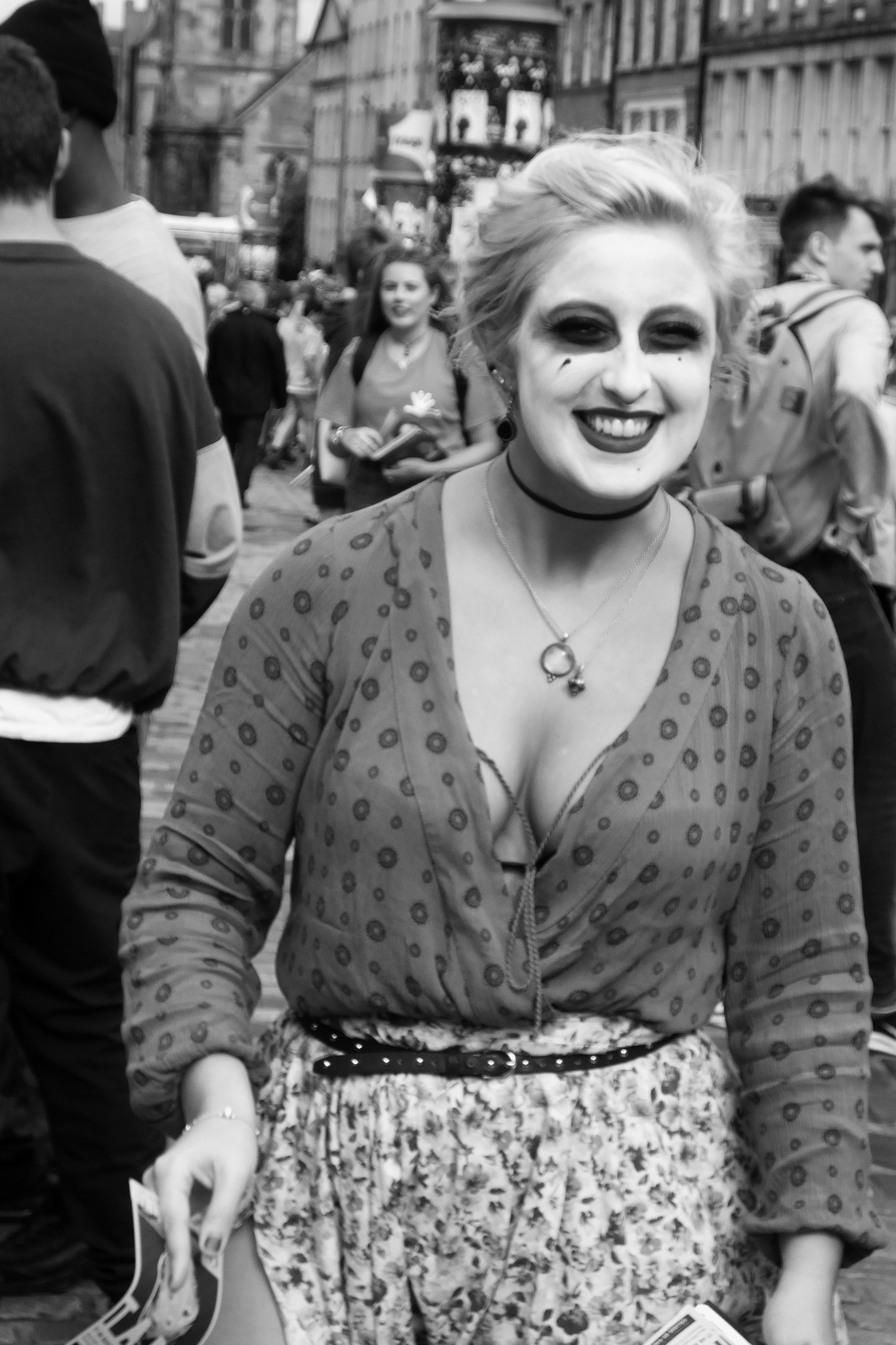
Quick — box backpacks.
[684,289,866,567]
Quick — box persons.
[698,175,895,997]
[0,0,243,1309]
[187,254,330,510]
[122,134,882,1344]
[319,245,506,513]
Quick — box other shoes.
[0,1210,129,1295]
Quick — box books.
[371,425,447,474]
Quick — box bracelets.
[184,1109,259,1136]
[335,426,352,447]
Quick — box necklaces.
[392,333,427,356]
[484,453,671,693]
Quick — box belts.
[299,1018,683,1081]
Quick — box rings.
[388,477,393,482]
